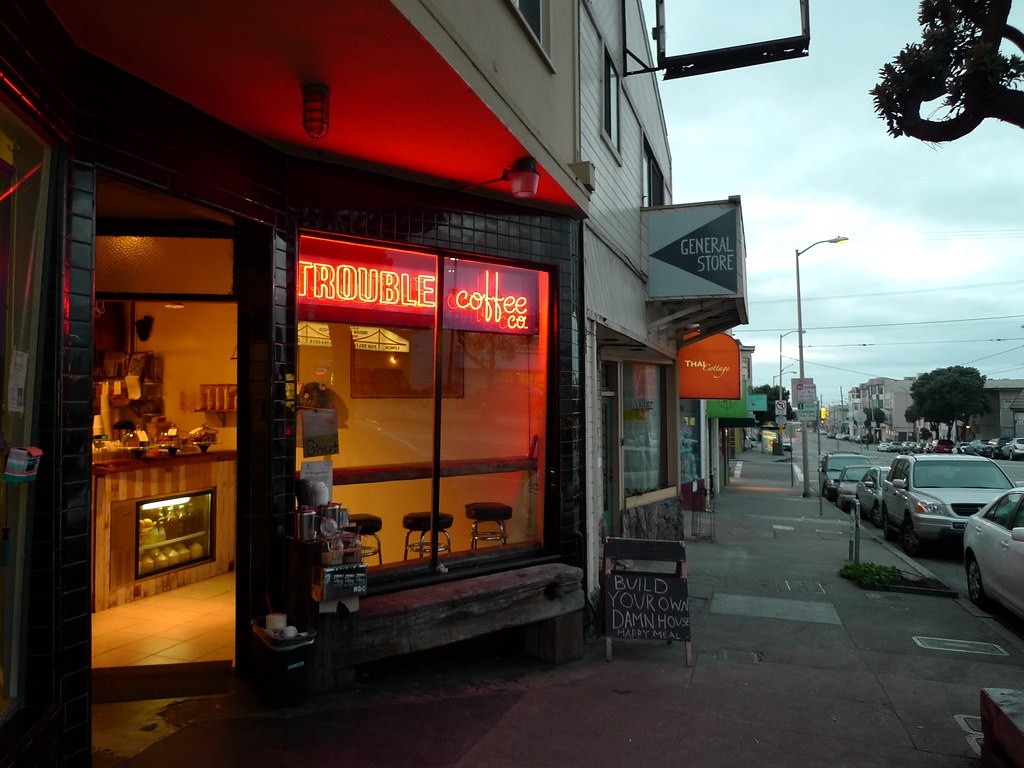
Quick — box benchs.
[296,561,590,694]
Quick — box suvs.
[886,442,902,452]
[929,439,955,453]
[882,451,1024,554]
[964,439,990,456]
[982,437,1012,459]
[1000,438,1024,460]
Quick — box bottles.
[139,502,203,575]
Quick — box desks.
[295,454,538,489]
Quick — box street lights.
[773,372,796,389]
[775,331,806,443]
[796,236,848,499]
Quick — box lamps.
[455,154,541,200]
[300,81,331,139]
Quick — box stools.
[345,513,382,565]
[402,511,453,561]
[464,501,513,550]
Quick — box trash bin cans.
[773,439,783,455]
[252,617,317,708]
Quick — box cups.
[294,510,318,543]
[283,626,298,640]
[266,613,287,631]
[318,502,340,533]
[308,481,330,507]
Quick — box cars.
[817,451,872,499]
[964,487,1024,619]
[834,464,880,510]
[782,438,791,449]
[855,467,891,528]
[819,429,869,443]
[908,441,932,453]
[746,436,758,448]
[898,442,912,453]
[877,442,890,452]
[951,442,969,454]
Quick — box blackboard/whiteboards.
[595,536,693,642]
